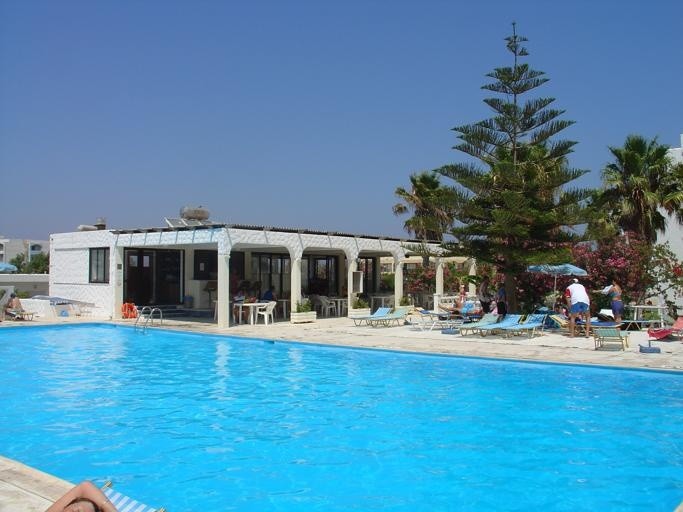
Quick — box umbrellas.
[0,262,17,272]
[528,260,587,304]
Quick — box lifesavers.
[122,303,138,318]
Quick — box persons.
[4,293,22,314]
[563,277,590,337]
[231,279,277,325]
[593,276,624,322]
[436,274,507,325]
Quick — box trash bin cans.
[183,295,193,308]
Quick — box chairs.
[5,304,37,321]
[99,480,166,512]
